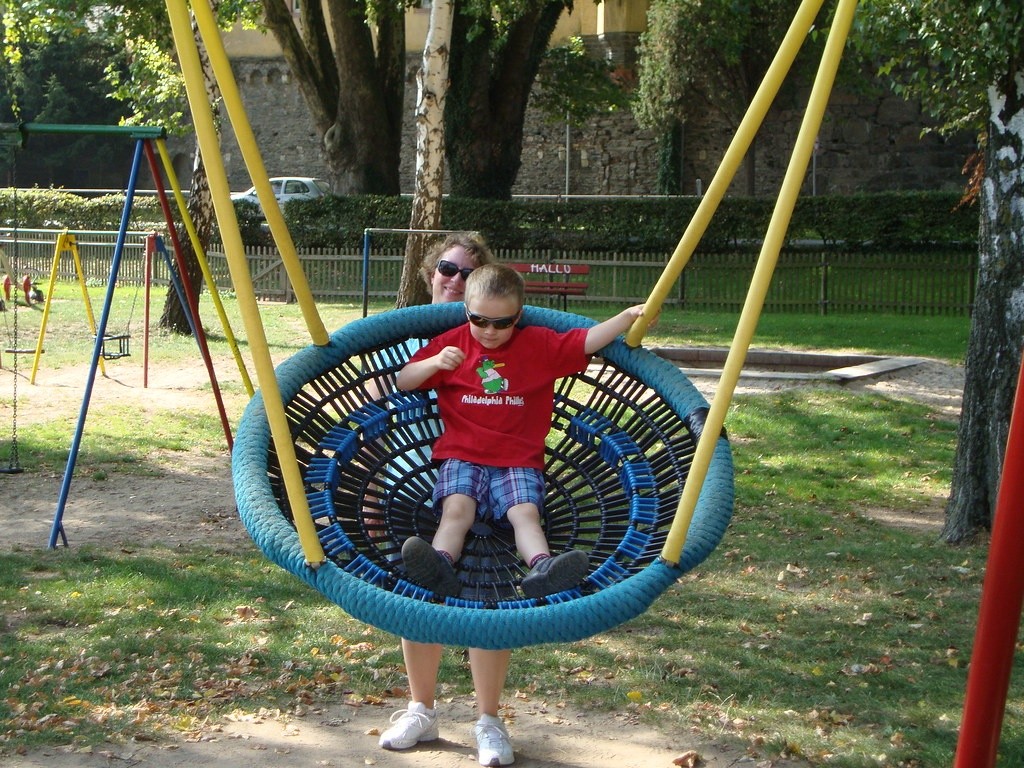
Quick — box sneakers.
[469,713,515,766]
[379,701,439,750]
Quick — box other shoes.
[520,551,589,598]
[401,536,461,598]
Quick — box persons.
[395,263,660,597]
[378,229,515,767]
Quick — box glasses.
[467,309,521,330]
[438,260,474,281]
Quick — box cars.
[230,176,331,222]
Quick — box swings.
[74,242,147,362]
[0,243,45,355]
[164,0,863,653]
[0,128,27,477]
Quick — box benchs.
[495,264,591,313]
